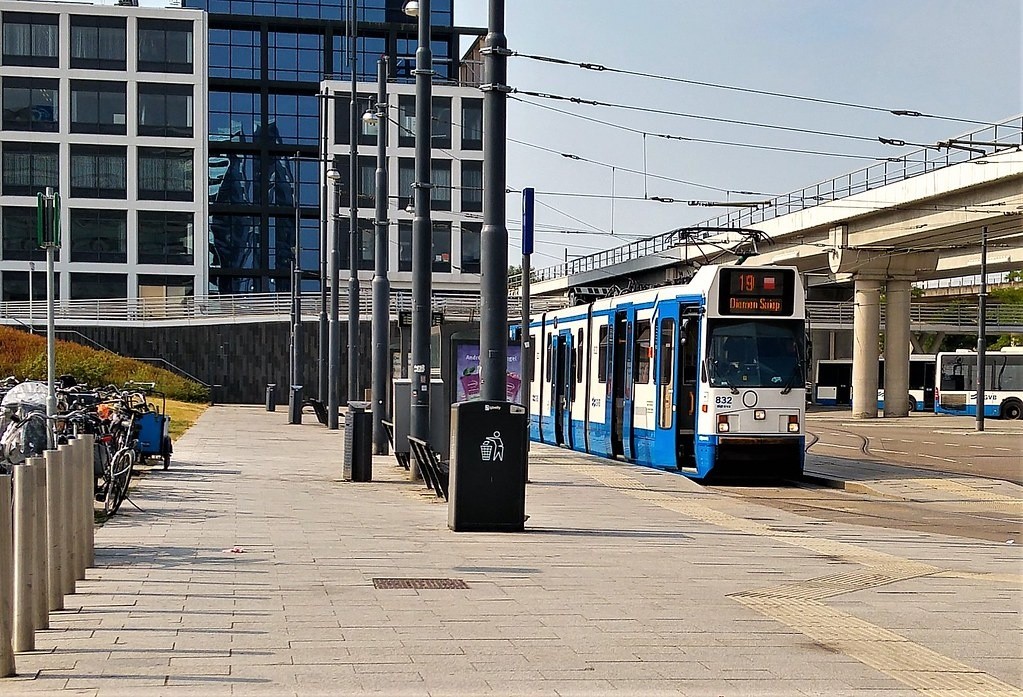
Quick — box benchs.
[309,398,328,425]
[407,436,450,502]
[382,419,411,470]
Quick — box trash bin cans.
[288,386,304,423]
[266,384,276,411]
[448,400,529,533]
[343,400,373,483]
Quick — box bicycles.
[0,371,174,517]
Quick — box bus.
[502,225,811,486]
[933,345,1023,421]
[810,353,936,412]
